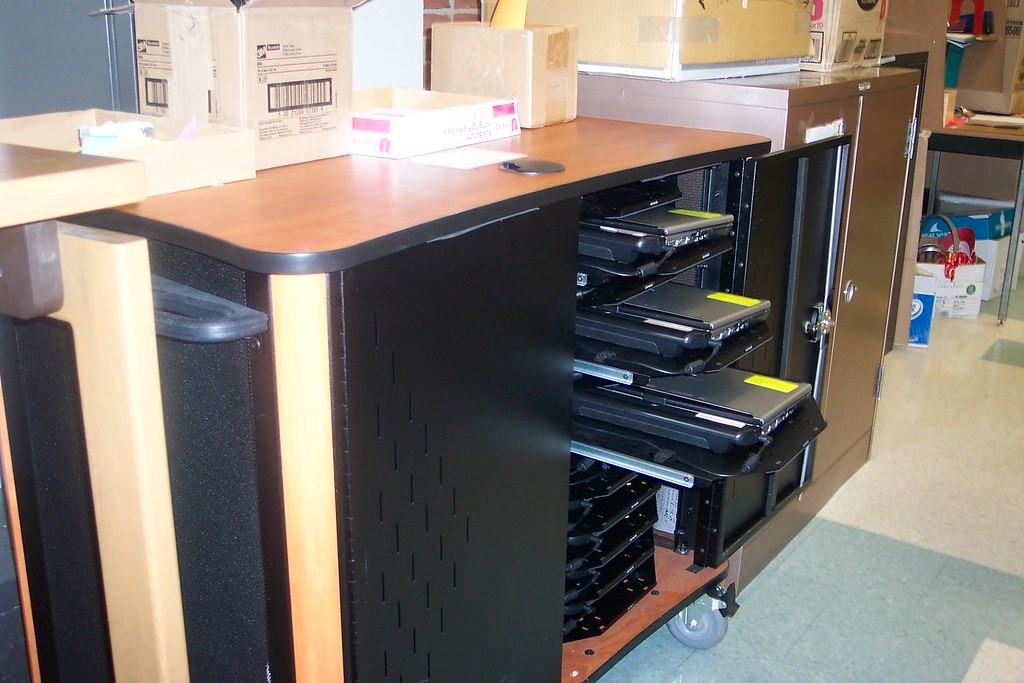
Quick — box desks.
[927,114,1024,324]
[0,142,144,683]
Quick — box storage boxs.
[909,209,1024,349]
[135,1,522,171]
[801,0,888,72]
[430,23,579,129]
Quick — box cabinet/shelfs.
[57,116,772,682]
[581,68,922,595]
